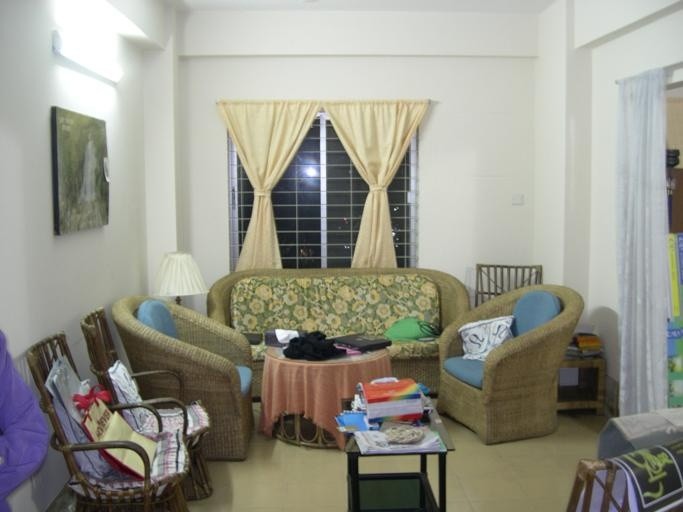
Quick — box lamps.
[153,253,210,306]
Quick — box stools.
[557,357,606,415]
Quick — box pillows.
[457,315,515,362]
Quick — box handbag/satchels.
[73,384,158,481]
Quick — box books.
[567,331,602,360]
[334,410,446,455]
[329,332,392,353]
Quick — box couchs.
[207,267,469,396]
[111,297,252,460]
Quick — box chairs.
[27,331,192,508]
[81,306,213,501]
[436,284,584,446]
